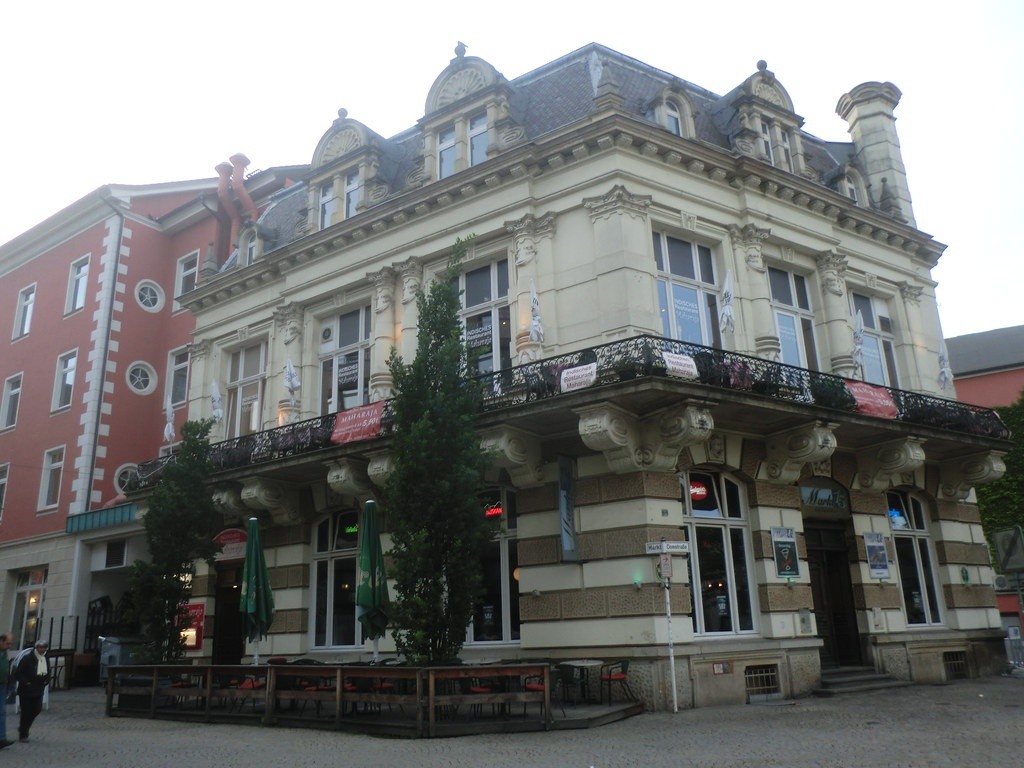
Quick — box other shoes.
[0,739,14,748]
[18,727,30,743]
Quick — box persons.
[17,638,52,743]
[0,632,15,748]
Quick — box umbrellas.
[210,379,223,432]
[239,517,276,666]
[528,279,543,360]
[164,395,175,454]
[354,499,392,662]
[720,269,736,350]
[937,346,954,398]
[851,309,865,382]
[283,360,302,417]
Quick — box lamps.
[485,501,503,517]
[633,580,641,589]
[532,589,541,598]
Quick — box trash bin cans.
[99,635,150,680]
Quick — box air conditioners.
[992,574,1009,591]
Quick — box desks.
[461,658,502,665]
[322,660,360,665]
[560,660,604,706]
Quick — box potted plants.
[161,636,193,665]
[752,371,778,397]
[649,362,667,377]
[615,357,637,381]
[808,379,856,409]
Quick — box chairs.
[524,372,556,402]
[165,656,634,722]
